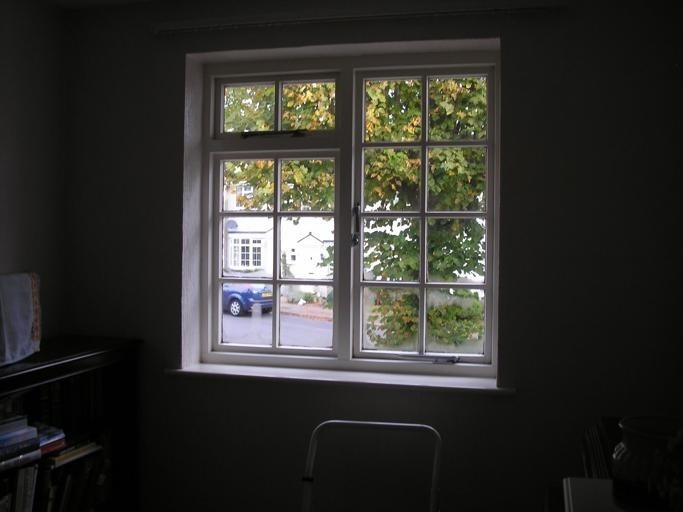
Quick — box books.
[0,407,106,512]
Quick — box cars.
[223,283,273,317]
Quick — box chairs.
[299,417,443,512]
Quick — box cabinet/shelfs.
[0,335,144,511]
